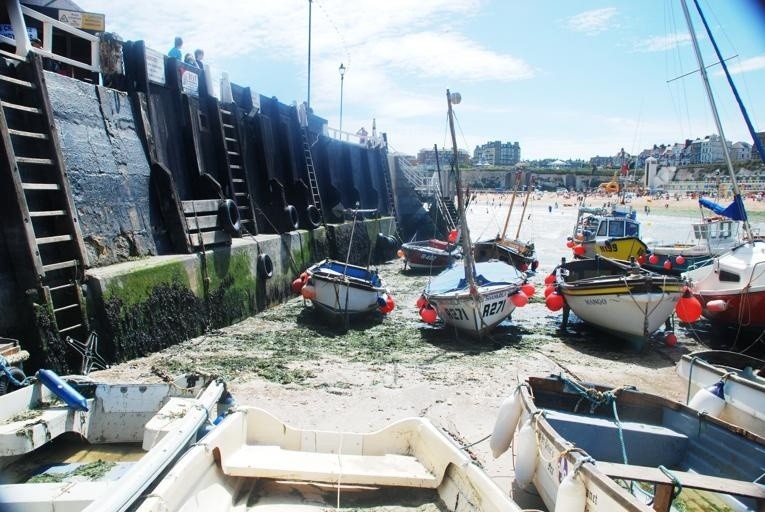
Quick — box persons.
[356,127,369,144]
[469,187,765,216]
[168,35,183,60]
[303,101,313,113]
[188,48,208,69]
